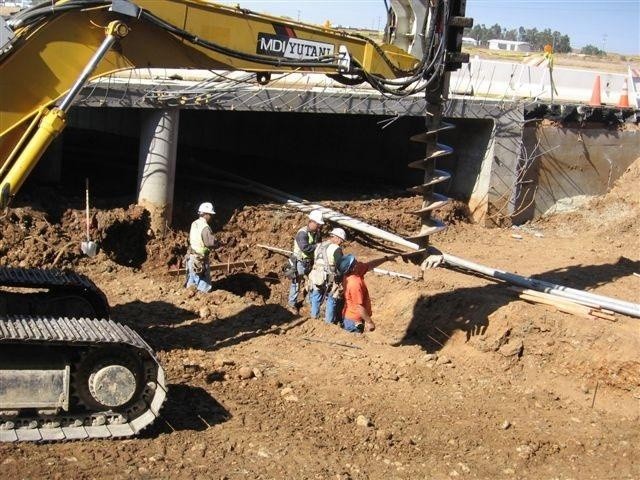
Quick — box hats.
[336,254,355,274]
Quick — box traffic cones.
[614,77,633,109]
[587,75,603,108]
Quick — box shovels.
[81,178,97,257]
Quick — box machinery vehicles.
[0,0,474,443]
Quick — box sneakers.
[286,304,299,315]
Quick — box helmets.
[197,202,217,215]
[308,210,325,225]
[328,228,347,242]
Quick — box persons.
[337,253,398,336]
[286,210,324,309]
[308,227,347,325]
[185,202,227,292]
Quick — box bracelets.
[385,255,390,261]
